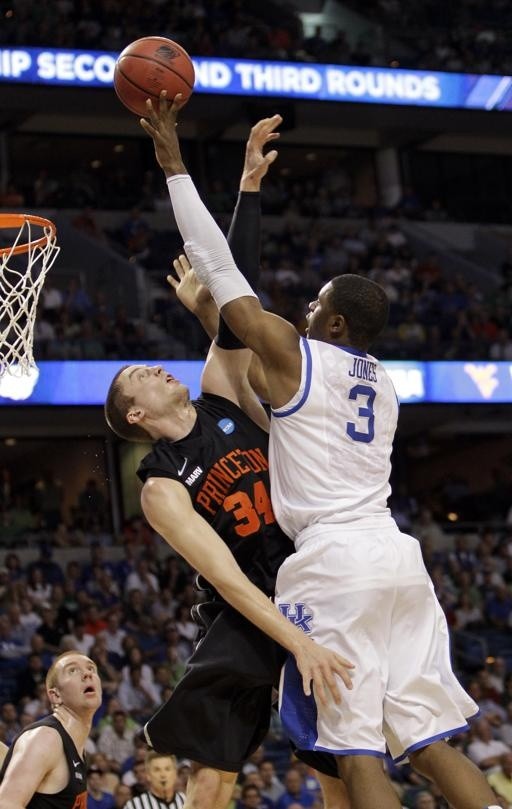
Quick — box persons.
[2,477,203,809]
[230,711,324,808]
[2,1,510,77]
[141,90,501,809]
[209,173,511,362]
[2,167,209,361]
[107,116,355,809]
[383,499,511,809]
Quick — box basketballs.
[114,36,194,117]
